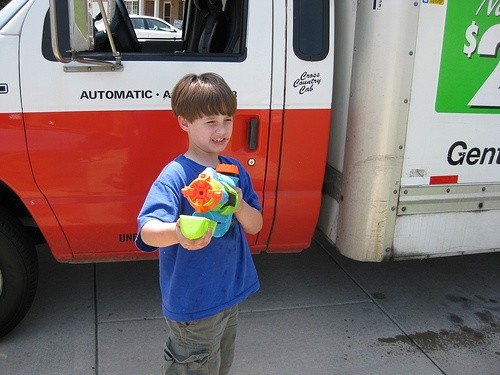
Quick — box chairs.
[194,0,225,54]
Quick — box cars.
[97,13,183,42]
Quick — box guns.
[180,162,241,239]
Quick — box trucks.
[0,0,500,339]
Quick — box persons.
[128,71,265,374]
